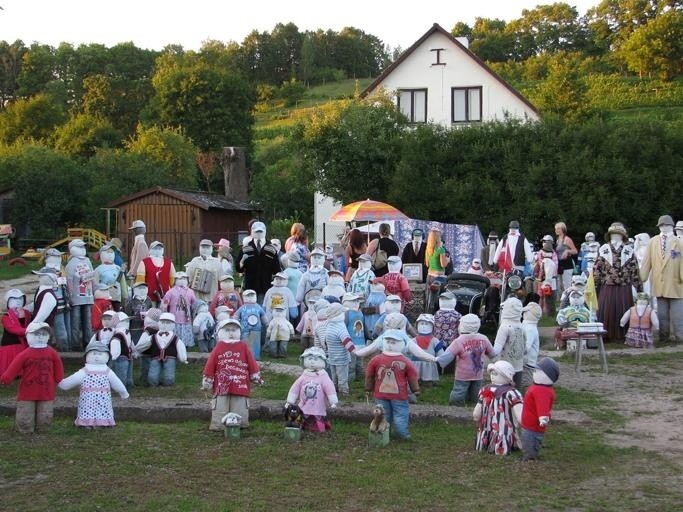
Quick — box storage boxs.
[576,322,604,332]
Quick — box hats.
[508,220,520,228]
[487,231,499,238]
[355,254,371,260]
[673,219,683,230]
[251,221,268,233]
[149,240,164,248]
[342,291,365,303]
[634,292,650,304]
[328,269,344,277]
[309,249,325,256]
[602,221,629,241]
[500,297,522,319]
[5,270,292,363]
[214,237,230,248]
[313,299,330,313]
[525,357,560,382]
[29,235,123,275]
[368,275,388,288]
[415,313,435,329]
[300,347,327,360]
[385,294,401,302]
[308,296,320,303]
[386,255,400,262]
[125,219,145,230]
[567,286,585,297]
[289,251,300,262]
[326,302,349,319]
[439,289,456,299]
[654,214,675,227]
[541,230,601,263]
[199,239,213,247]
[521,302,542,319]
[412,229,424,239]
[458,313,480,334]
[487,360,516,384]
[471,259,482,267]
[382,328,408,347]
[571,274,586,286]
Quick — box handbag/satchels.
[370,242,389,272]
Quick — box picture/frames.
[402,263,422,281]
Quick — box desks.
[574,330,608,373]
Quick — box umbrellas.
[323,198,410,246]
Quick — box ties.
[660,235,668,258]
[256,240,262,252]
